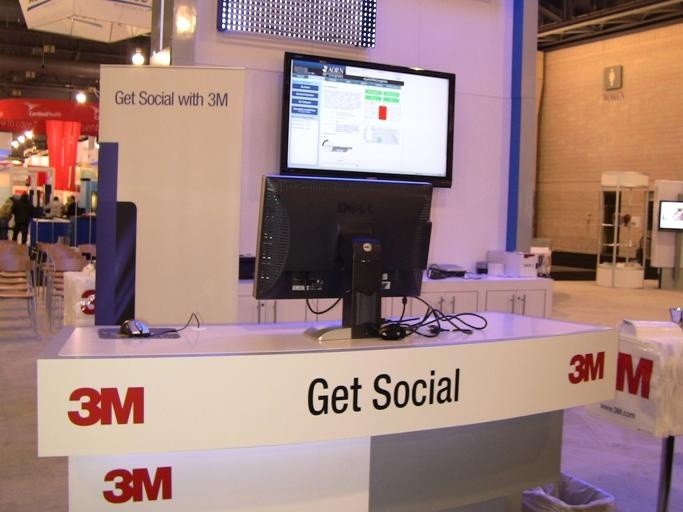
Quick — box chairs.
[0,241,96,332]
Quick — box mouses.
[121,320,150,337]
[379,324,401,339]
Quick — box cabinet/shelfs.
[595,171,650,289]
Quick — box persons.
[0,195,16,240]
[67,196,78,218]
[12,193,34,244]
[46,197,63,218]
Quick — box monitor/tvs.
[280,52,455,188]
[657,200,683,231]
[253,174,433,343]
